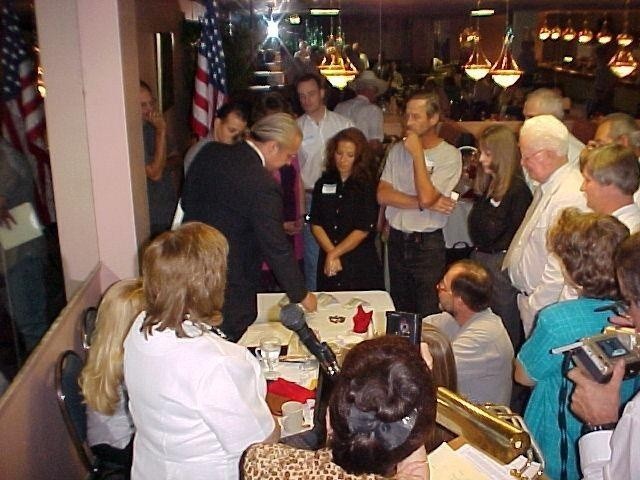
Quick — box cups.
[279,401,304,434]
[253,337,282,375]
[450,191,459,201]
[299,361,318,389]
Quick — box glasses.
[439,276,452,295]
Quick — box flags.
[192,9,231,140]
[0,0,55,225]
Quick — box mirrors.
[0,0,103,410]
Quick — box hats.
[347,70,390,97]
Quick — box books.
[0,201,45,251]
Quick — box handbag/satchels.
[446,241,472,263]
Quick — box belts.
[473,243,501,255]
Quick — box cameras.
[552,333,640,383]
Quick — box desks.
[237,290,396,438]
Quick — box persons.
[239,334,438,480]
[139,39,464,343]
[76,277,147,480]
[122,219,282,480]
[420,40,638,479]
[0,136,50,349]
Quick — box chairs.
[82,305,98,353]
[54,350,129,479]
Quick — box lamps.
[462,1,493,82]
[316,1,359,92]
[538,1,636,80]
[488,1,524,88]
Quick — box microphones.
[280,303,342,382]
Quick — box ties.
[501,187,542,272]
